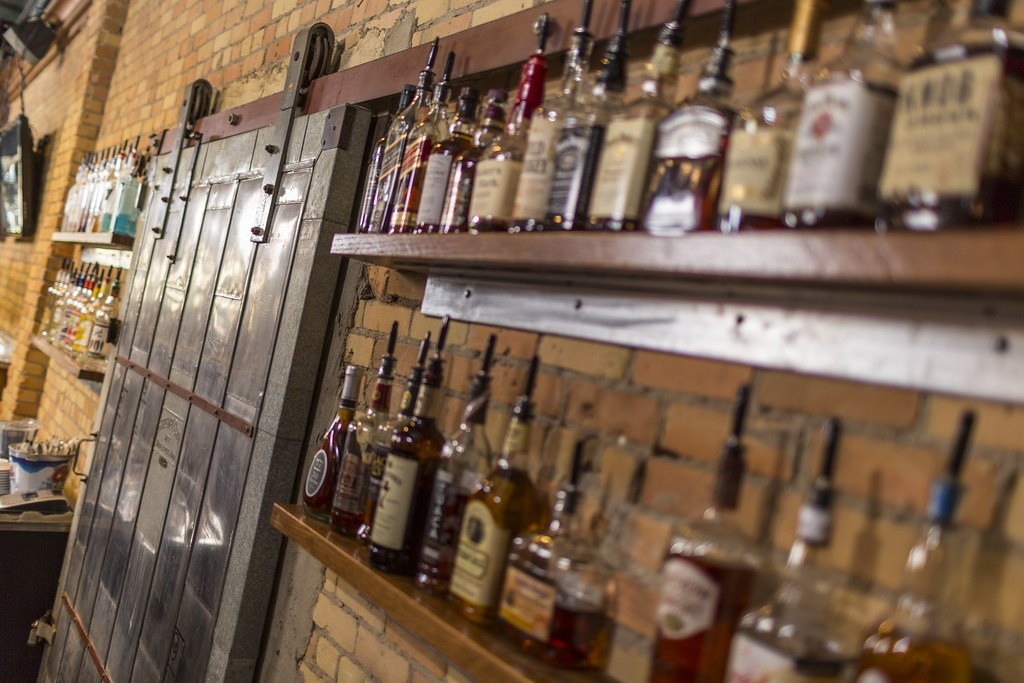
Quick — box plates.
[0,458,10,495]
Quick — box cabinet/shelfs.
[269,231,1024,683]
[0,506,75,683]
[31,231,135,383]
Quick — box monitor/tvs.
[0,114,39,239]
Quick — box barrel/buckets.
[0,417,40,459]
[8,442,75,494]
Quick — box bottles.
[59,137,152,239]
[350,0,1023,238]
[39,258,122,362]
[300,320,628,673]
[649,383,977,683]
